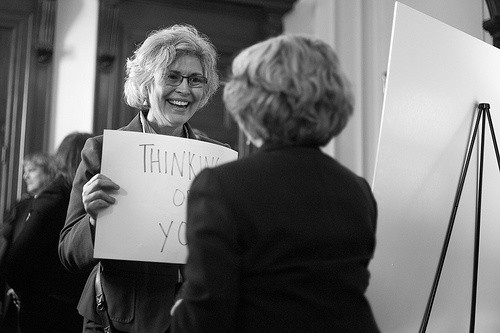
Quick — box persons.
[9,132,94,333]
[168,34,381,333]
[0,151,54,333]
[57,27,231,333]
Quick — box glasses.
[163,70,207,90]
